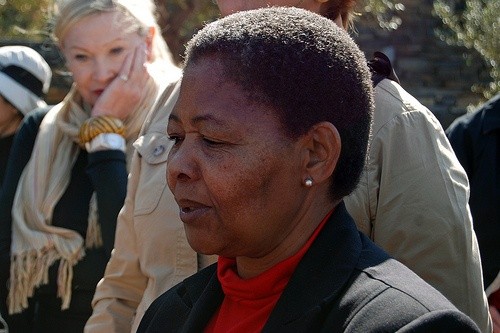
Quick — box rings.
[118,73,128,81]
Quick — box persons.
[83,0,493,333]
[0,0,172,333]
[0,45,52,192]
[135,6,481,333]
[444,92,500,333]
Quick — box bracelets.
[80,114,127,149]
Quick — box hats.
[0,45,52,116]
